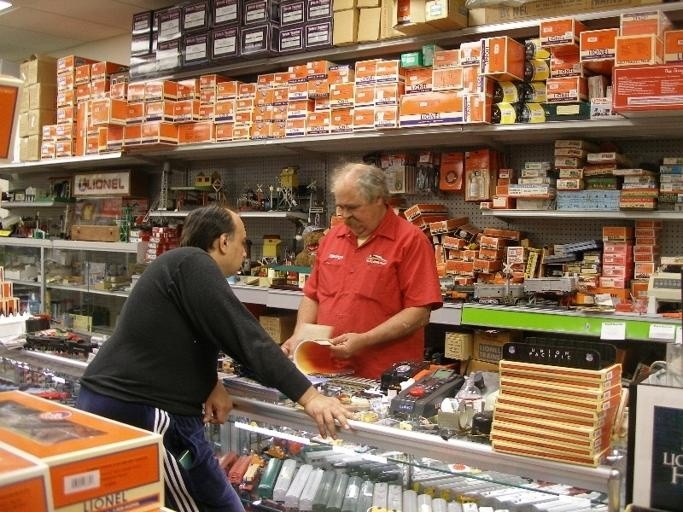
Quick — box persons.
[277,162,443,382]
[74,204,358,511]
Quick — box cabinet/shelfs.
[0,150,162,343]
[1,348,631,512]
[130,1,683,350]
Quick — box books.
[286,322,356,378]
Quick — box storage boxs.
[16,54,129,161]
